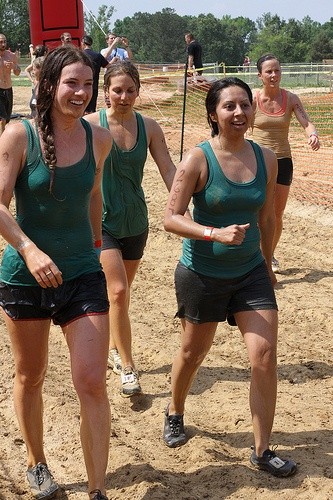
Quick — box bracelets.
[310,134,318,138]
[95,240,102,247]
[204,227,213,240]
[96,249,100,255]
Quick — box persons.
[184,33,203,76]
[251,54,320,272]
[237,57,249,72]
[25,45,48,117]
[81,36,110,114]
[61,32,71,44]
[0,45,110,500]
[83,62,193,397]
[0,34,21,136]
[163,77,296,476]
[100,33,132,108]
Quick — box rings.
[46,271,51,275]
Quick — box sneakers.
[272,258,281,273]
[163,402,187,448]
[25,463,60,499]
[107,348,123,374]
[121,365,143,398]
[250,443,298,477]
